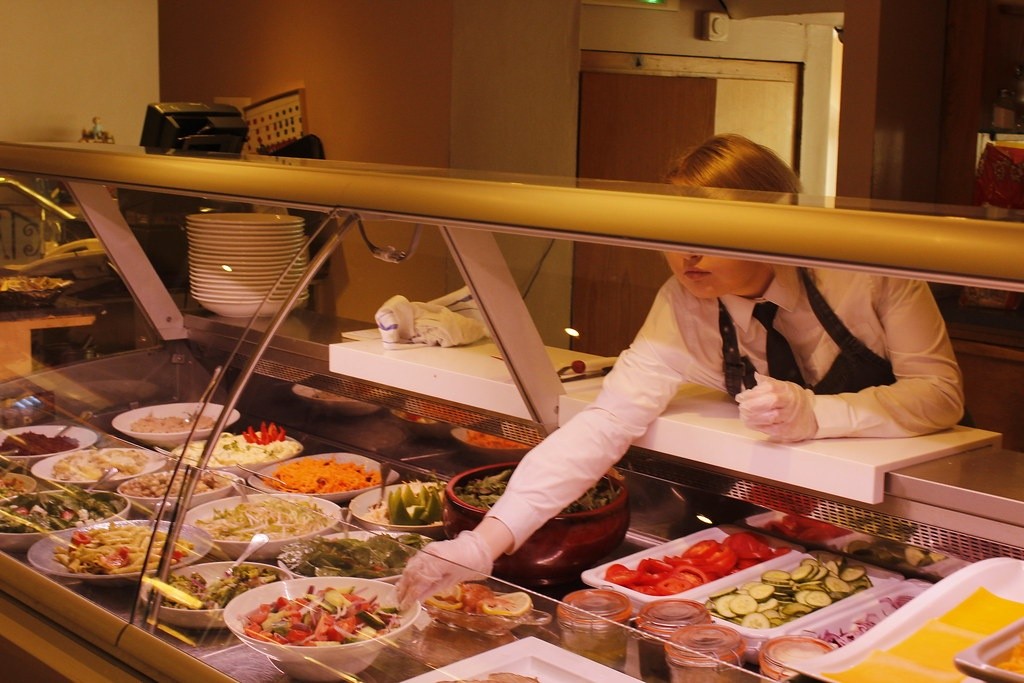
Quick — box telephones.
[18,238,107,278]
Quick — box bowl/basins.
[441,461,630,588]
[223,576,421,681]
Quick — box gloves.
[736,371,817,442]
[397,530,493,611]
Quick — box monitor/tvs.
[267,133,330,283]
[140,98,249,154]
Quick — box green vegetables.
[278,528,435,580]
[448,464,624,515]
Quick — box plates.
[182,493,343,561]
[111,403,241,450]
[30,448,167,488]
[348,481,448,532]
[954,614,1024,683]
[139,561,295,629]
[421,583,553,636]
[184,212,309,318]
[277,530,437,585]
[0,472,37,504]
[26,519,213,588]
[116,469,240,511]
[0,425,99,460]
[247,452,400,505]
[0,276,76,305]
[168,431,304,479]
[580,524,939,665]
[0,489,131,552]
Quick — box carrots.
[265,458,384,494]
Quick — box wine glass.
[337,507,352,532]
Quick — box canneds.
[625,598,711,683]
[756,634,834,683]
[554,584,631,670]
[661,623,747,683]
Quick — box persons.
[394,133,967,614]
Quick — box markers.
[572,357,618,373]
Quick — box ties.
[752,301,805,388]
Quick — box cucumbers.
[702,553,873,631]
[0,486,118,534]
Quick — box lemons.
[481,591,532,616]
[423,584,463,610]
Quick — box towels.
[376,286,493,349]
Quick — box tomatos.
[245,592,375,645]
[605,532,792,595]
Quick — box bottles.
[637,599,712,683]
[759,635,836,683]
[556,589,632,674]
[664,624,747,683]
